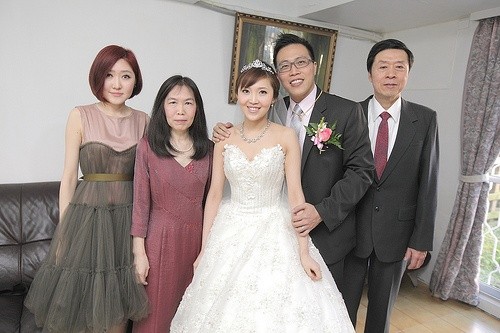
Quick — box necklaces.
[290,89,322,122]
[169,140,195,152]
[240,118,270,143]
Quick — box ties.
[372,112,391,179]
[291,104,302,138]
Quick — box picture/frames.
[227,10,339,106]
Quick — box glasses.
[277,57,315,73]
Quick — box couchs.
[0,180,84,333]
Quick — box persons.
[170,58,356,333]
[131,74,216,333]
[213,33,375,328]
[342,38,439,333]
[23,44,151,332]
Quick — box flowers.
[302,117,346,155]
